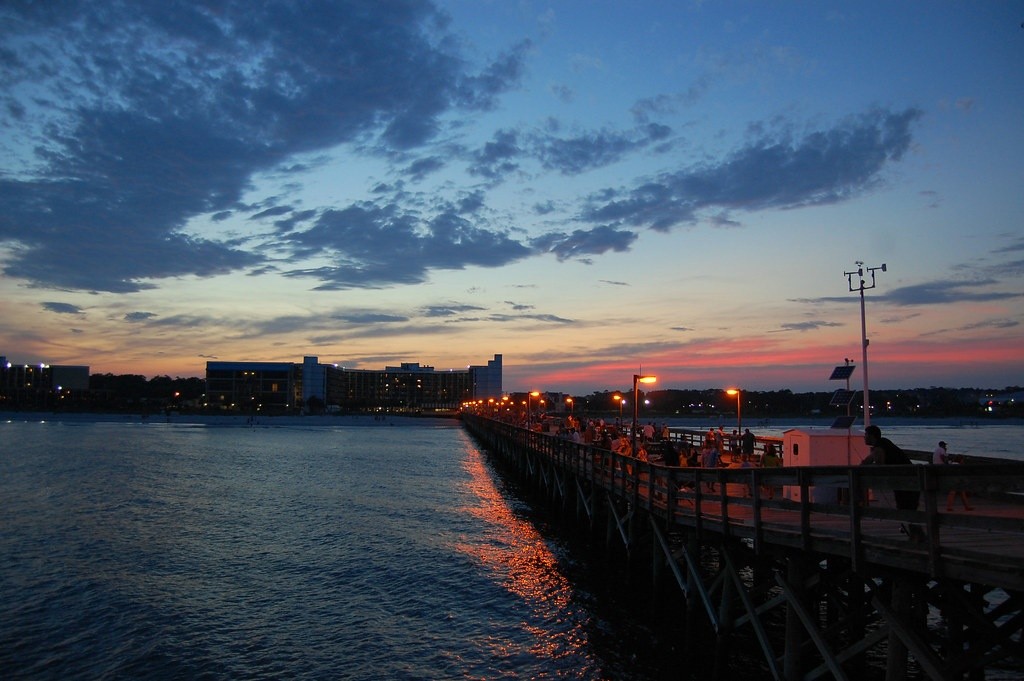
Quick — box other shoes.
[965,506,975,511]
[710,487,715,492]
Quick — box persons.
[946,454,974,513]
[471,407,757,496]
[759,444,780,500]
[933,441,948,463]
[859,425,926,543]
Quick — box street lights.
[527,389,539,433]
[613,393,624,430]
[633,373,658,458]
[726,386,742,449]
[566,397,574,417]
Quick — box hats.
[939,441,948,446]
